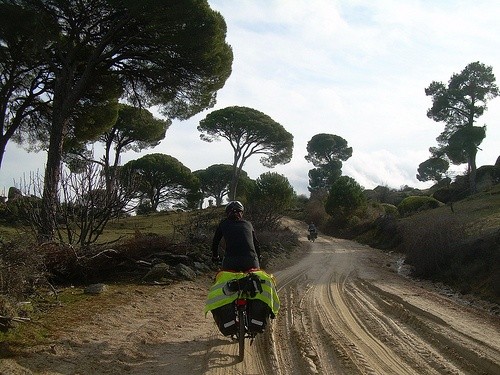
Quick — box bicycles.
[211,254,252,361]
[309,229,316,242]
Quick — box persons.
[215,202,263,272]
[308,222,316,233]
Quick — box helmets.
[225,201,244,212]
